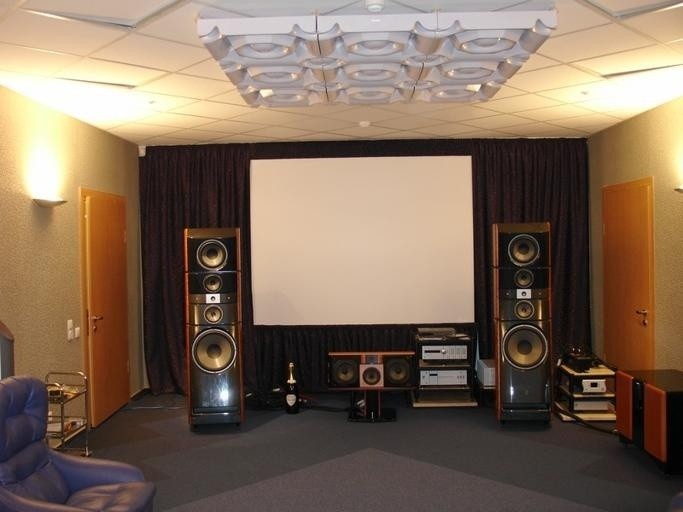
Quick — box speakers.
[491,222,554,425]
[184,229,245,432]
[328,351,416,391]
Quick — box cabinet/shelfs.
[328,332,480,422]
[45,372,92,458]
[555,362,683,480]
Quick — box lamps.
[34,198,68,208]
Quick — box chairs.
[0,375,157,512]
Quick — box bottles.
[285,362,299,415]
